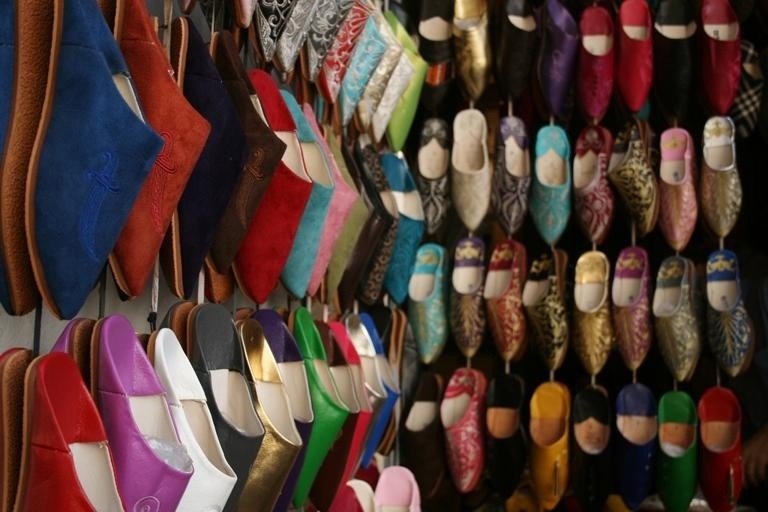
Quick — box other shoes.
[0,0,426,512]
[403,0,756,512]
